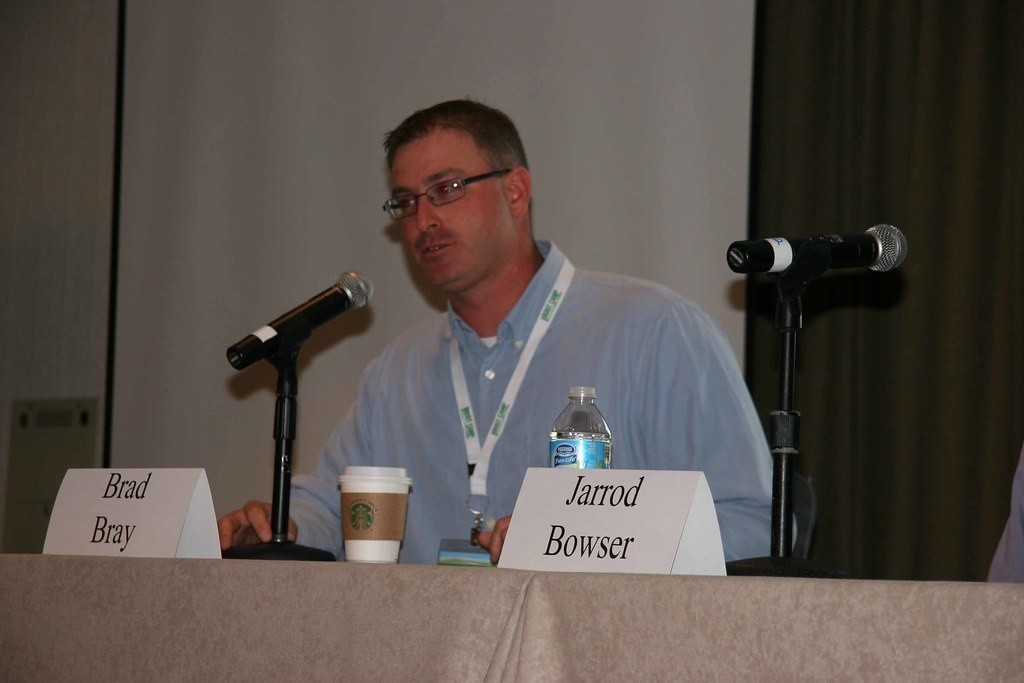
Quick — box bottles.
[548,386,612,470]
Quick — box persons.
[218,100,793,566]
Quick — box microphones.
[226,271,374,370]
[727,224,907,271]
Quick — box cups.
[338,465,412,563]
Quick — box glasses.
[382,168,513,219]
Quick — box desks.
[0,554,1024,683]
